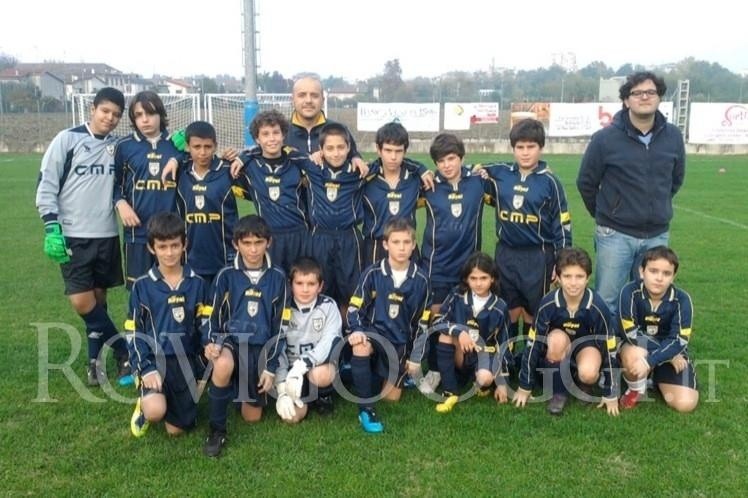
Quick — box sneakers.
[547,392,569,416]
[417,369,442,396]
[472,380,491,398]
[130,396,152,439]
[340,362,353,385]
[204,423,228,458]
[315,385,335,417]
[435,390,461,414]
[113,345,133,380]
[402,376,417,389]
[579,384,594,407]
[357,405,385,434]
[86,361,107,387]
[619,383,648,410]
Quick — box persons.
[32,86,189,390]
[114,76,625,459]
[614,245,699,412]
[575,71,686,391]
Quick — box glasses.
[626,87,659,99]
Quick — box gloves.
[42,220,73,266]
[169,127,189,153]
